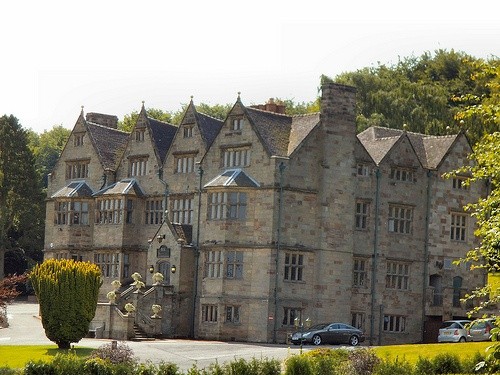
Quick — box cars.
[438,320,474,344]
[292,322,365,346]
[466,318,496,341]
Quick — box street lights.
[293,318,312,355]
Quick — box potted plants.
[106,292,117,303]
[152,304,162,316]
[152,272,163,284]
[125,303,135,315]
[111,280,122,292]
[135,281,145,291]
[132,272,142,284]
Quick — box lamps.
[171,265,176,273]
[149,265,154,273]
